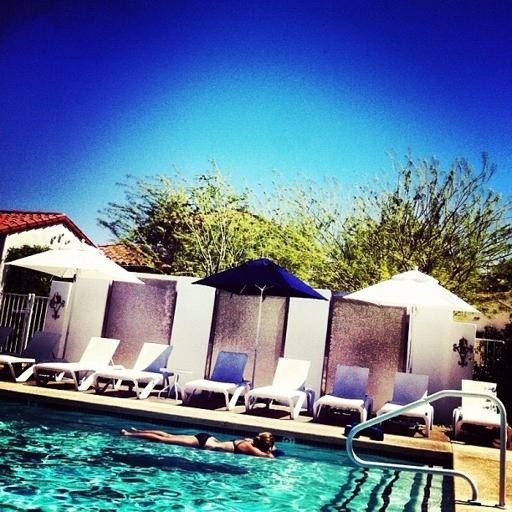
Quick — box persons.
[117,425,279,459]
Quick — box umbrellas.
[342,269,483,373]
[191,259,328,390]
[6,239,146,362]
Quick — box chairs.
[183,350,435,439]
[451,379,503,440]
[0,331,181,401]
